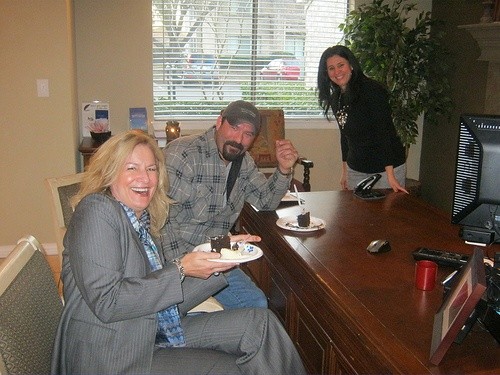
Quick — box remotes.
[411,246,470,267]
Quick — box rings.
[214,272,219,275]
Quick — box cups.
[414,260,439,292]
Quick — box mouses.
[366,239,391,253]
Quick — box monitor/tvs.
[449,112,500,243]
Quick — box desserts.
[210,234,242,257]
[296,211,310,227]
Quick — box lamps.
[166,121,180,141]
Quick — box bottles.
[165,121,180,140]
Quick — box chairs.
[0,234,225,375]
[50,174,85,272]
[221,110,314,192]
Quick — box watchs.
[277,164,293,175]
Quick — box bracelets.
[169,258,185,283]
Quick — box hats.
[222,100,260,134]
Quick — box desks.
[78,137,166,173]
[232,186,500,375]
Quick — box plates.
[192,242,263,263]
[276,215,325,232]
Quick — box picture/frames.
[429,246,487,366]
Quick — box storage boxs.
[81,102,110,137]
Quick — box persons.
[317,46,410,194]
[52,130,310,375]
[151,101,297,308]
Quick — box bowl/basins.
[90,130,111,143]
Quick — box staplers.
[354,173,385,199]
